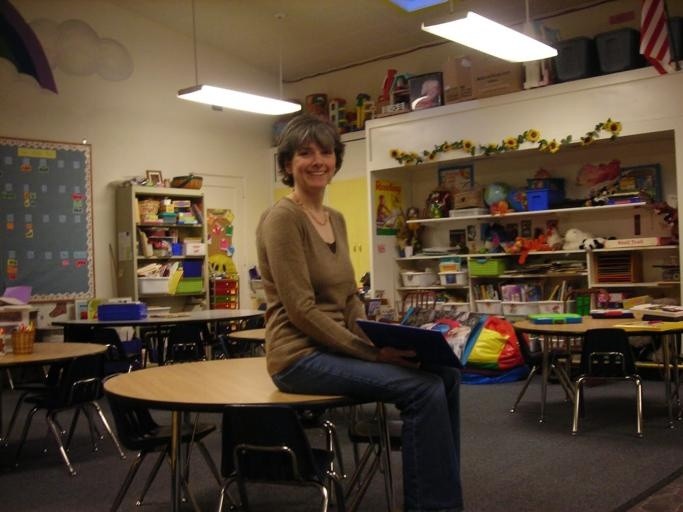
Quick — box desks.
[226,328,265,356]
[512,316,683,420]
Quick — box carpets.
[0,378,683,512]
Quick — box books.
[474,280,580,300]
[423,246,459,256]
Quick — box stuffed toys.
[480,225,617,264]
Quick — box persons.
[255,111,463,511]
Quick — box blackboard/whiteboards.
[0,137,96,302]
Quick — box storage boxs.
[470,260,504,276]
[528,177,565,203]
[528,190,560,211]
[421,52,522,111]
[177,277,204,293]
[159,214,176,223]
[421,299,573,315]
[455,192,484,207]
[401,273,433,287]
[438,272,468,286]
[184,245,206,255]
[553,17,683,82]
[98,304,147,319]
[138,277,171,295]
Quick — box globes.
[484,182,510,206]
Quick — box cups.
[405,245,414,257]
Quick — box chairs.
[512,330,643,438]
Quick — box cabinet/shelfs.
[393,202,679,288]
[115,187,209,368]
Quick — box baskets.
[12,331,35,354]
[172,174,202,188]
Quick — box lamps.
[178,0,301,116]
[421,0,559,62]
